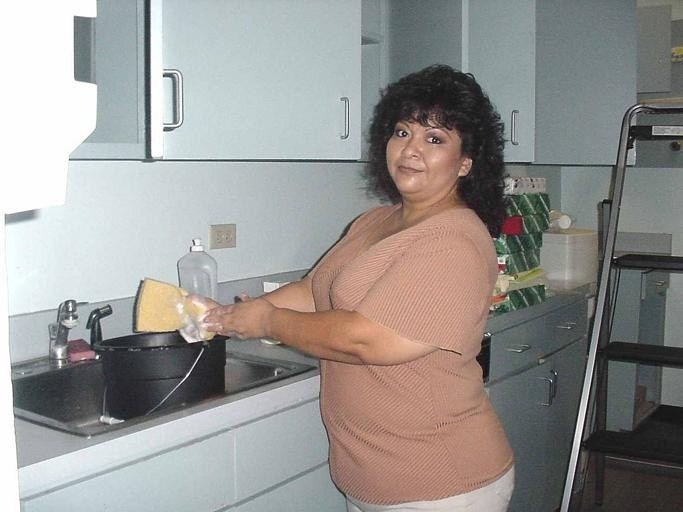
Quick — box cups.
[546,209,572,230]
[502,174,546,195]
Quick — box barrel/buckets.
[93,328,231,426]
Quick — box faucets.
[47,299,88,364]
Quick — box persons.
[183,62,516,512]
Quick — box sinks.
[12,348,318,439]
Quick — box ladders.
[559,99,683,512]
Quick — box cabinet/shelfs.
[488,295,593,512]
[633,268,670,433]
